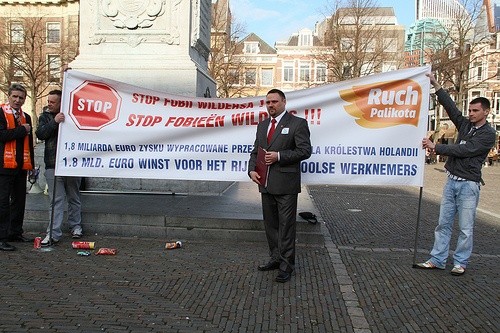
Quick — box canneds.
[72,241,96,249]
[33,236,42,249]
[165,243,176,249]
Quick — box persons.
[425,141,441,165]
[0,84,34,251]
[248,90,312,283]
[416,71,496,275]
[35,90,82,247]
[481,148,494,167]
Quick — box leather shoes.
[0,242,14,251]
[6,235,30,242]
[275,271,290,282]
[258,261,280,271]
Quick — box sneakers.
[41,232,58,245]
[451,265,465,275]
[412,260,437,269]
[72,228,82,239]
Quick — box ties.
[15,112,21,126]
[267,119,277,146]
